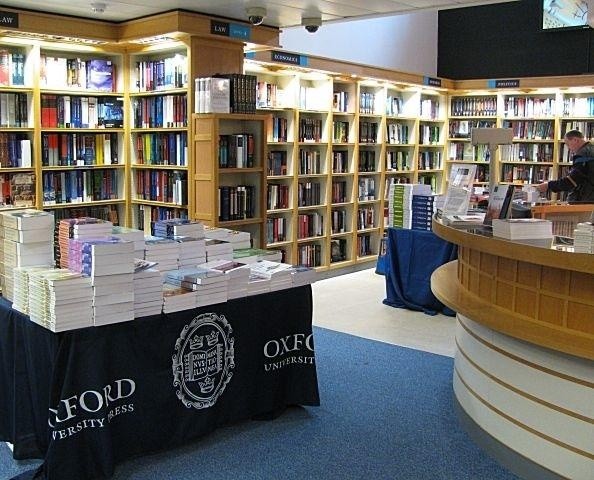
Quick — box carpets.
[1,326,523,480]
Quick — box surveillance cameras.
[301,18,322,32]
[246,7,267,26]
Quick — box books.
[0,208,322,333]
[134,168,187,231]
[1,170,119,226]
[268,149,321,176]
[194,72,258,114]
[265,113,320,143]
[451,97,593,255]
[445,212,486,226]
[217,133,254,169]
[0,93,124,168]
[132,95,189,167]
[217,184,256,222]
[0,49,190,93]
[332,91,446,265]
[268,182,321,210]
[255,80,320,111]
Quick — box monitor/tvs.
[539,0,594,33]
[511,202,533,219]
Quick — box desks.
[382,228,476,316]
[0,284,320,480]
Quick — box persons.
[531,130,593,204]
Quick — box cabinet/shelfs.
[2,39,40,215]
[191,113,267,255]
[126,43,192,242]
[36,42,128,230]
[244,58,449,280]
[497,90,563,201]
[446,89,499,189]
[556,88,593,201]
